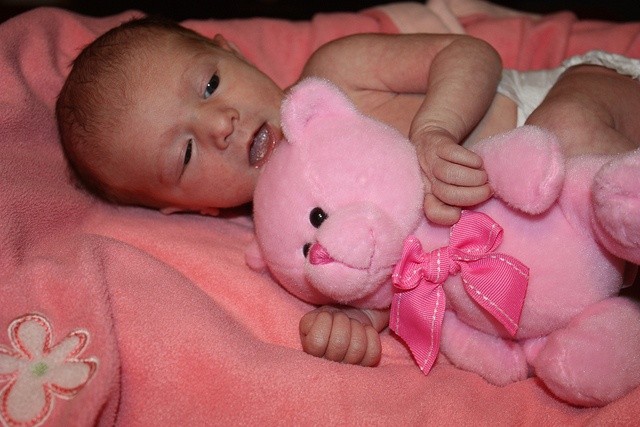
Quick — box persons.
[55,16,640,367]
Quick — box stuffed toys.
[245,76,639,408]
